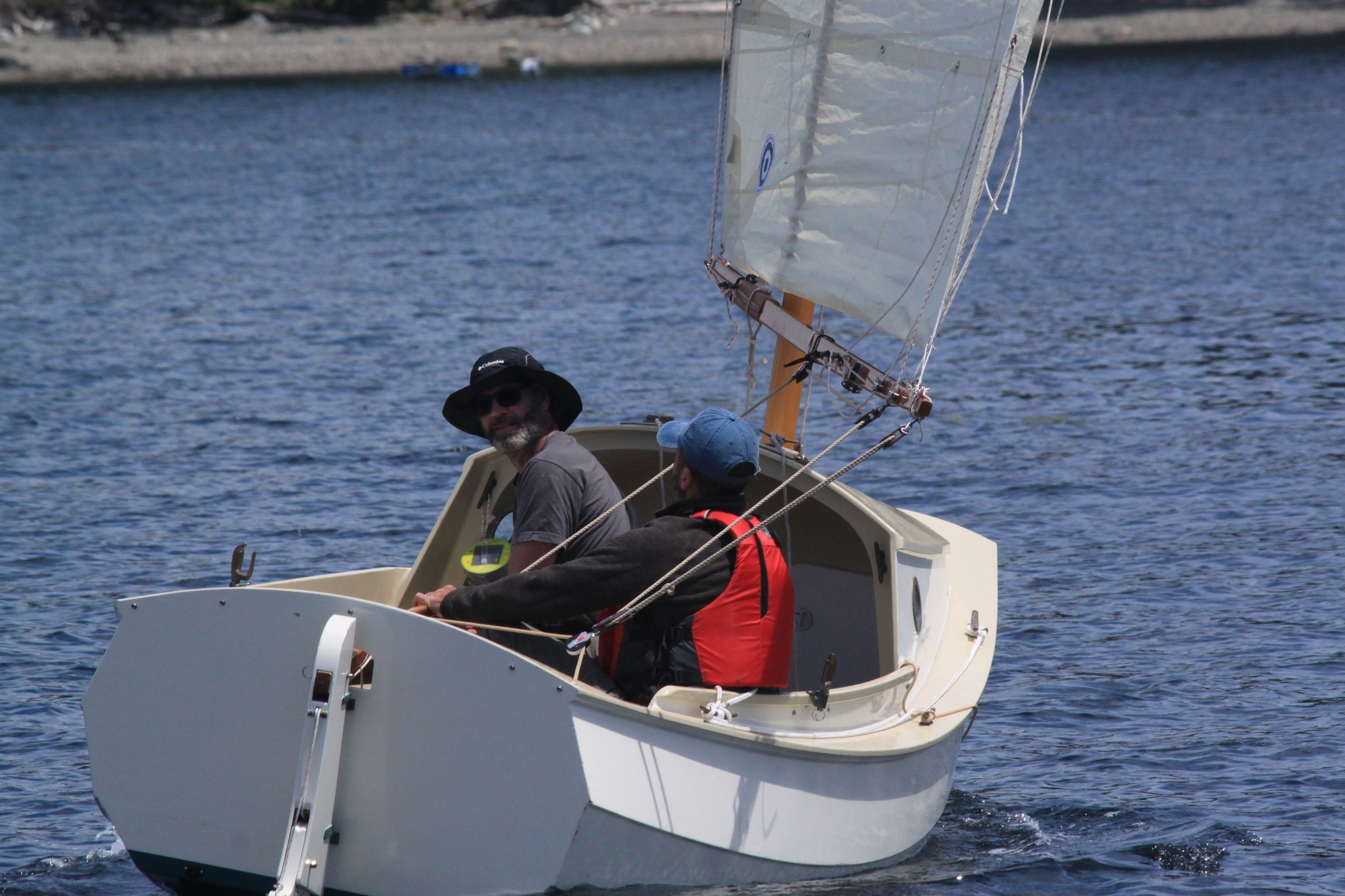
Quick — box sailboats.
[84,0,1058,895]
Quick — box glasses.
[471,381,542,416]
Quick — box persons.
[441,345,631,578]
[410,407,799,705]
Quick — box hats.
[442,347,582,439]
[656,406,761,484]
